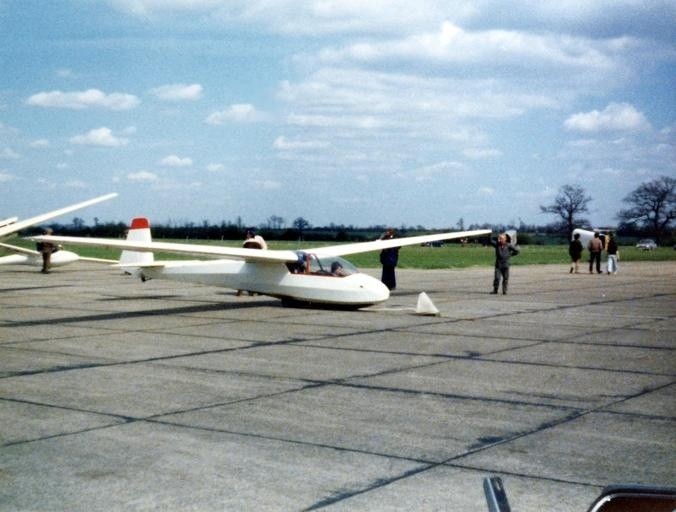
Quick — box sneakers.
[569,267,618,274]
[236,290,242,296]
[490,291,507,295]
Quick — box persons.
[379,231,401,291]
[329,261,345,278]
[568,234,585,273]
[607,236,621,275]
[40,228,63,275]
[486,232,521,294]
[250,227,267,295]
[235,231,262,297]
[587,233,604,274]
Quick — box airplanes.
[19,217,492,310]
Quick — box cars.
[636,239,657,250]
[422,241,444,247]
